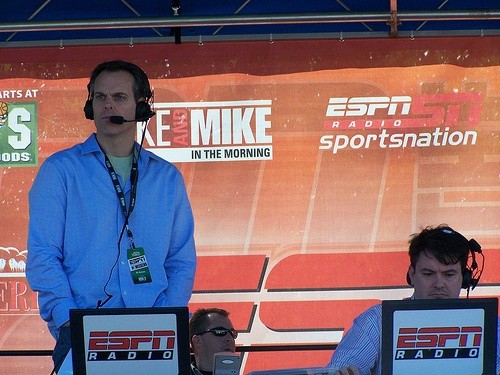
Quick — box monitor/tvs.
[382,298,498,375]
[69,307,191,375]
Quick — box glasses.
[197,327,238,338]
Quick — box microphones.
[469,238,485,290]
[110,116,148,125]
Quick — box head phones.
[84,73,156,122]
[407,229,476,290]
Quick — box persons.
[188,309,238,375]
[328,224,500,375]
[26,59,197,375]
